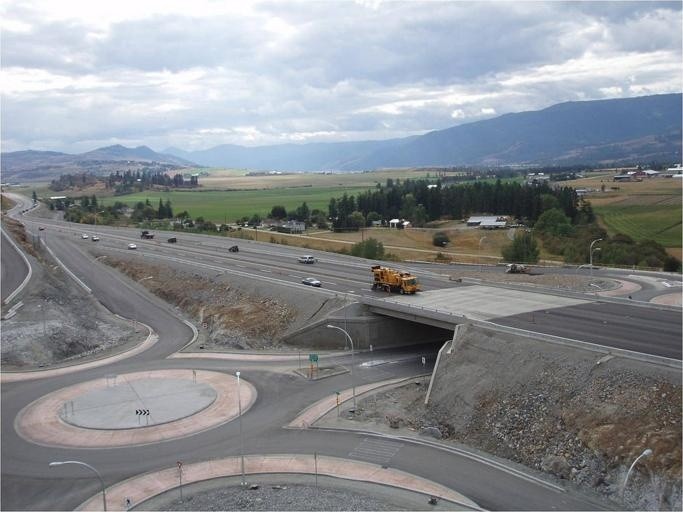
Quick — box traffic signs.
[135,409,149,415]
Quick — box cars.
[80,233,87,239]
[126,243,136,249]
[167,237,176,244]
[226,245,238,253]
[91,235,98,242]
[38,225,44,231]
[21,199,37,213]
[299,276,321,287]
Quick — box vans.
[297,254,314,265]
[432,236,450,247]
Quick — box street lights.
[620,448,652,510]
[342,289,356,351]
[133,276,153,336]
[589,238,603,290]
[91,254,107,299]
[478,235,487,264]
[234,371,246,490]
[47,461,106,511]
[575,262,591,293]
[94,211,104,244]
[326,325,356,413]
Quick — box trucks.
[139,230,153,239]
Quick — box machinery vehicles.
[369,266,420,294]
[504,264,527,274]
